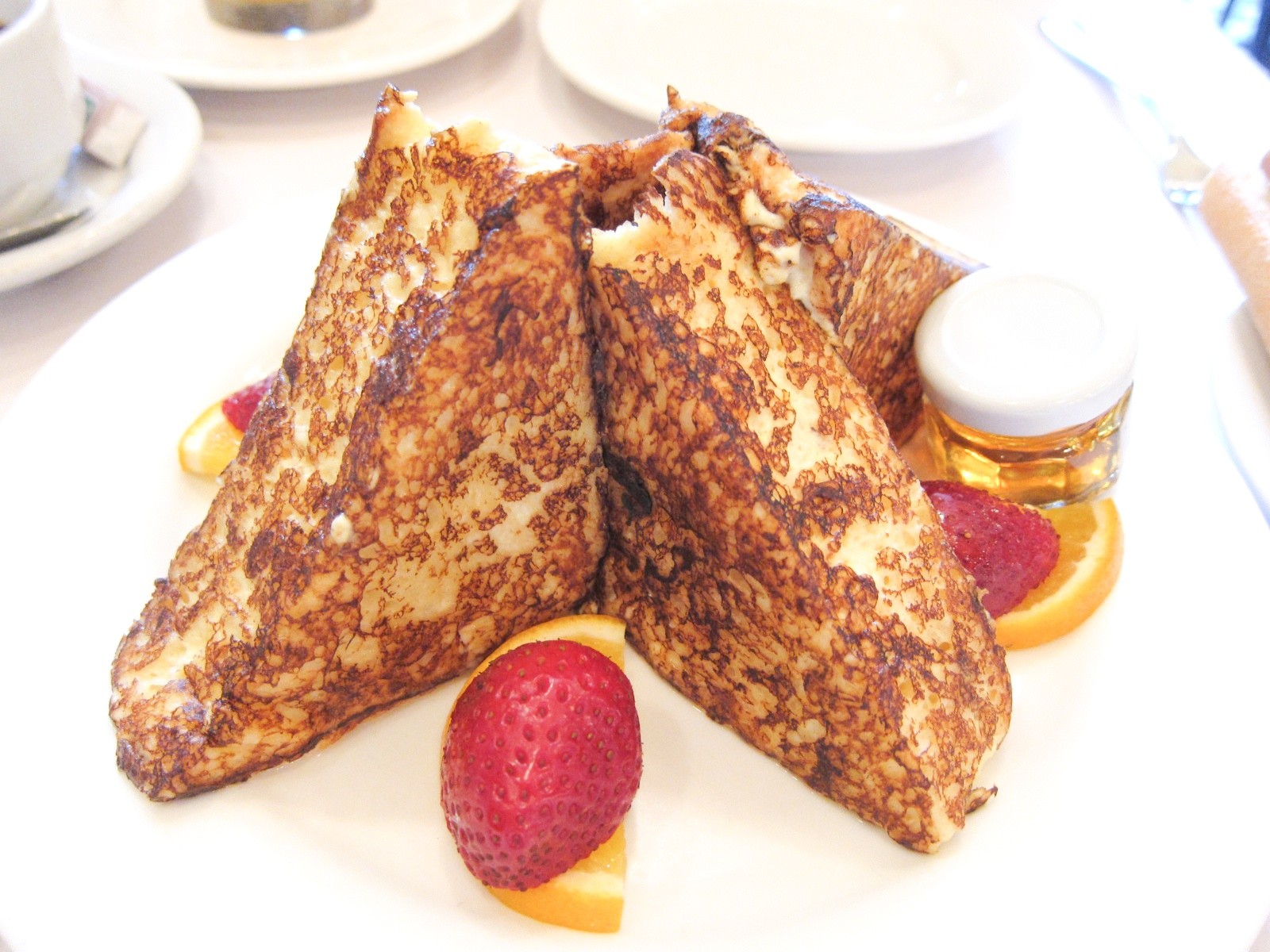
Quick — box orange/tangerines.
[440,614,625,933]
[984,500,1123,649]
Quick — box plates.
[0,63,209,292]
[66,0,523,90]
[5,172,1270,952]
[537,0,1051,157]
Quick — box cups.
[0,0,84,233]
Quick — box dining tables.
[0,0,1267,952]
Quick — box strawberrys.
[438,638,643,892]
[923,481,1061,619]
